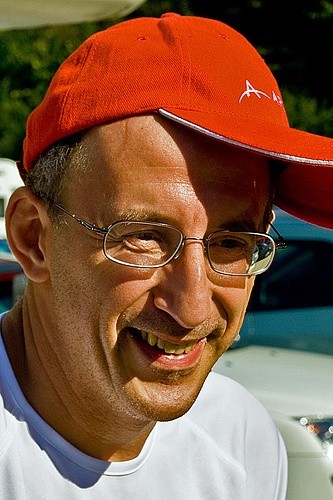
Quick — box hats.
[16,11,333,231]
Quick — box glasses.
[34,190,288,277]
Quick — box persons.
[0,12,333,500]
